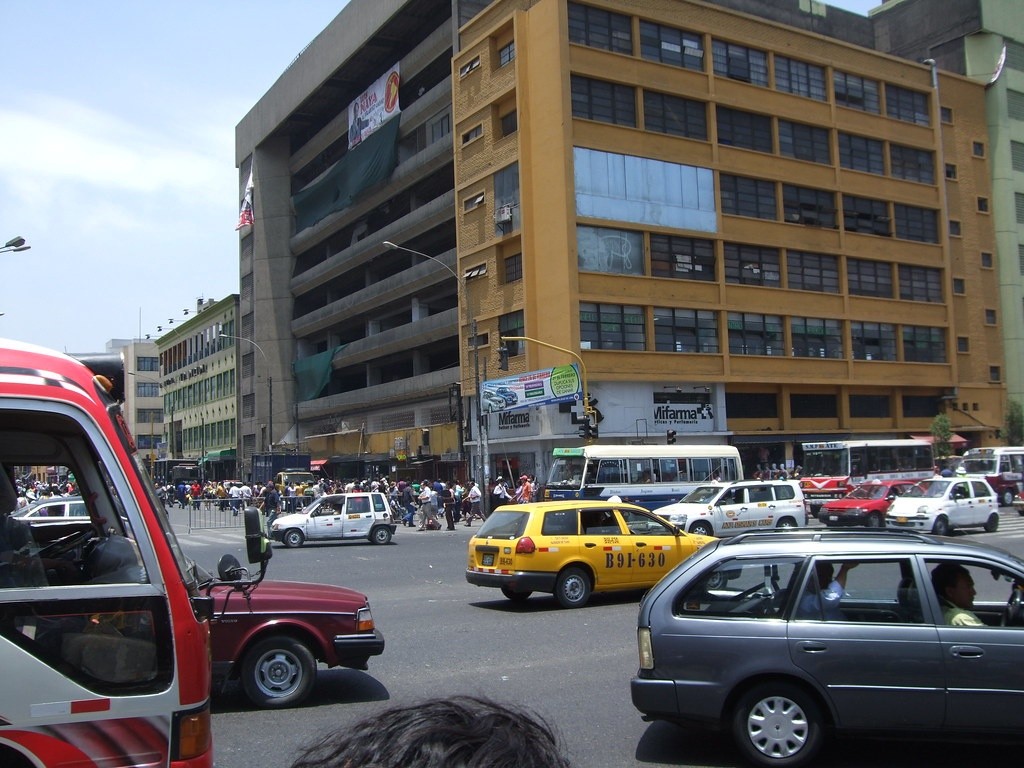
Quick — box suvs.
[646,476,809,538]
[629,522,1024,768]
[267,490,397,549]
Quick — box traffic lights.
[577,415,590,439]
[666,429,677,445]
[589,424,599,439]
[496,347,509,371]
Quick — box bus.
[799,437,936,518]
[0,338,277,768]
[542,445,745,523]
[956,447,1024,507]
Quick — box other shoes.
[465,524,470,527]
[409,524,416,527]
[401,519,406,526]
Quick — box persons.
[931,564,989,626]
[16,473,81,516]
[290,694,570,768]
[412,478,486,531]
[154,480,253,516]
[489,472,539,512]
[0,512,158,682]
[252,474,418,539]
[636,469,686,484]
[941,464,952,477]
[350,102,373,148]
[797,561,860,620]
[712,469,721,481]
[756,466,802,480]
[754,463,788,480]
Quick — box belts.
[421,502,431,505]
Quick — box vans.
[274,470,316,492]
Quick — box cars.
[9,495,132,533]
[816,477,930,528]
[464,494,744,609]
[181,552,385,711]
[884,474,1000,536]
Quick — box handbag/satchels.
[489,494,499,504]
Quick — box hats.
[956,467,966,473]
[942,470,952,476]
[390,481,396,488]
[519,476,527,481]
[496,477,502,481]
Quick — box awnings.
[909,434,968,444]
[200,448,236,462]
[310,460,329,477]
[327,451,396,465]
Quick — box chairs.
[897,577,914,619]
[908,580,924,621]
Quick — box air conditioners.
[494,206,511,225]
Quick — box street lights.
[219,333,273,455]
[126,372,176,459]
[382,240,487,521]
